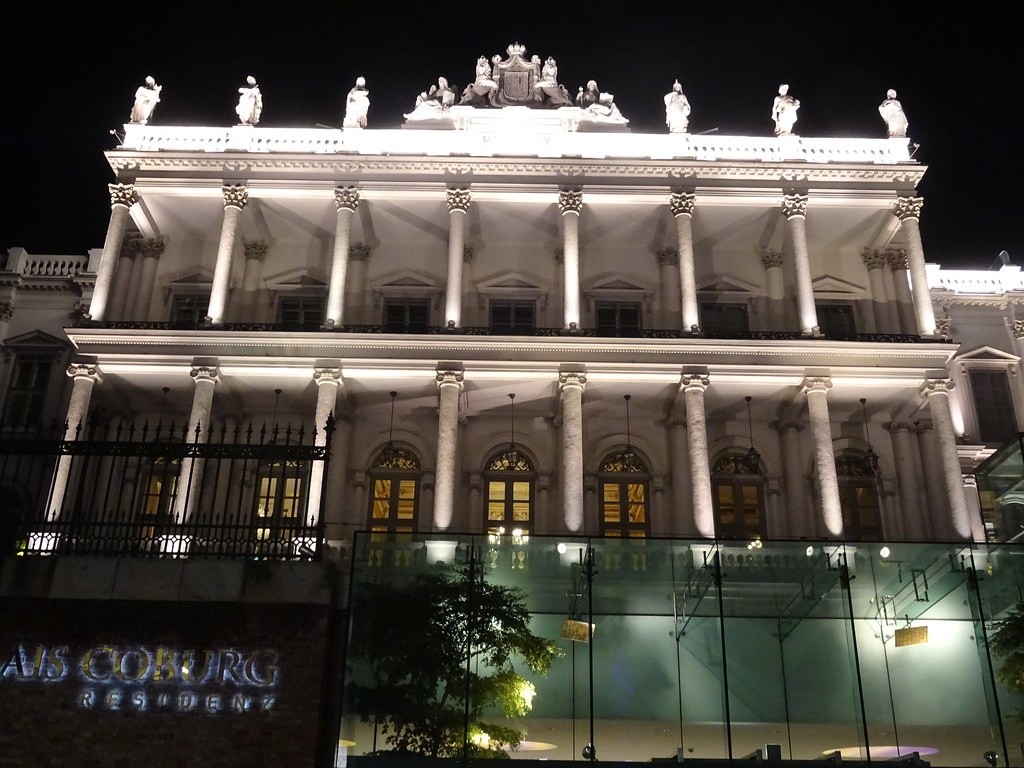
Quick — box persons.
[577,80,614,116]
[343,77,369,126]
[539,58,557,82]
[403,77,452,118]
[476,58,499,89]
[235,76,262,125]
[664,84,690,132]
[879,89,909,136]
[773,84,800,135]
[130,76,161,122]
